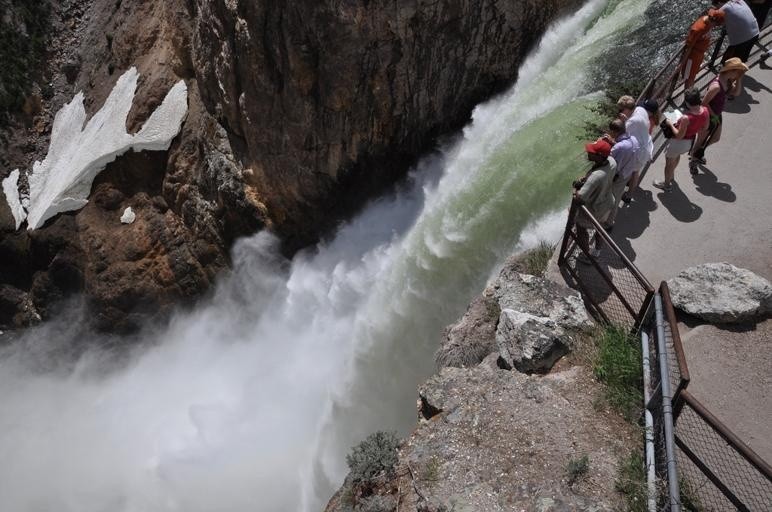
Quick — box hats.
[719,56,749,74]
[585,139,611,159]
[637,98,658,112]
[708,6,726,23]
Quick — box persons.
[572,139,619,267]
[637,56,750,195]
[617,94,655,204]
[707,0,761,103]
[595,116,642,234]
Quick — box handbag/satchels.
[660,118,673,139]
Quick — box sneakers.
[651,154,708,192]
[577,190,636,267]
